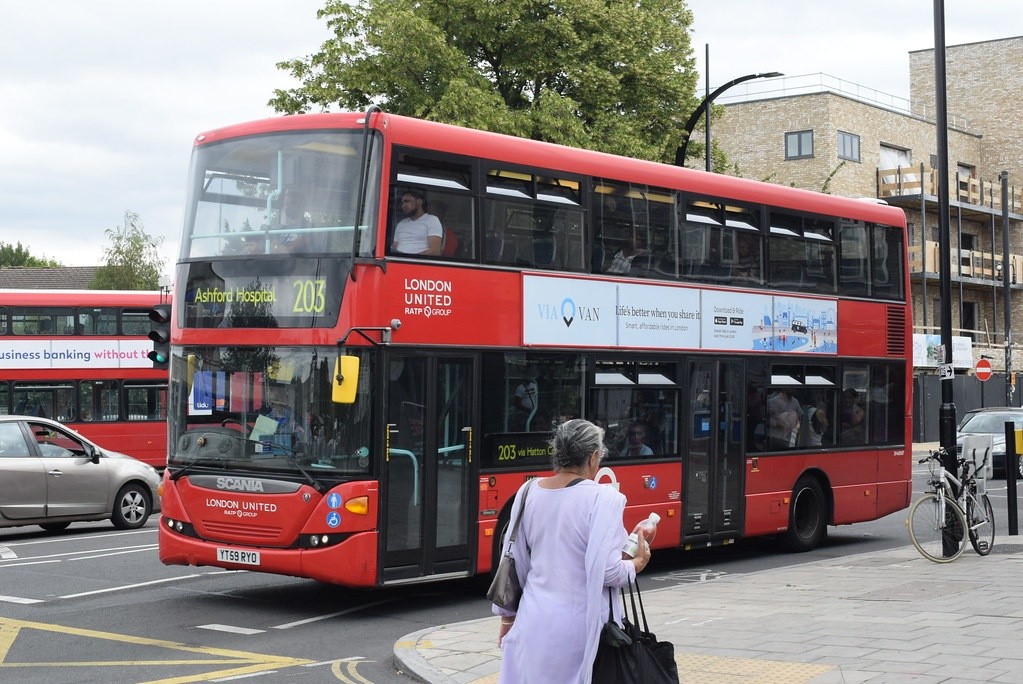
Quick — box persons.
[838,388,864,440]
[618,422,654,456]
[72,322,84,335]
[81,409,92,422]
[601,225,681,280]
[390,191,443,257]
[730,238,758,288]
[801,388,829,447]
[486,419,651,684]
[40,319,53,334]
[241,192,316,254]
[514,371,538,415]
[251,385,312,455]
[744,378,806,447]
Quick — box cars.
[957,406,1023,479]
[0,415,163,530]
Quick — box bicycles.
[907,446,995,563]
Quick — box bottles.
[623,512,661,560]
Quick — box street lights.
[674,71,785,165]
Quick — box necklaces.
[559,471,578,475]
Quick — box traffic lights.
[147,305,170,372]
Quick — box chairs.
[442,218,896,299]
[36,319,85,335]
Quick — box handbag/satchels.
[592,573,679,684]
[486,478,537,612]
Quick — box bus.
[0,288,264,472]
[153,107,915,589]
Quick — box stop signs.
[975,361,994,383]
